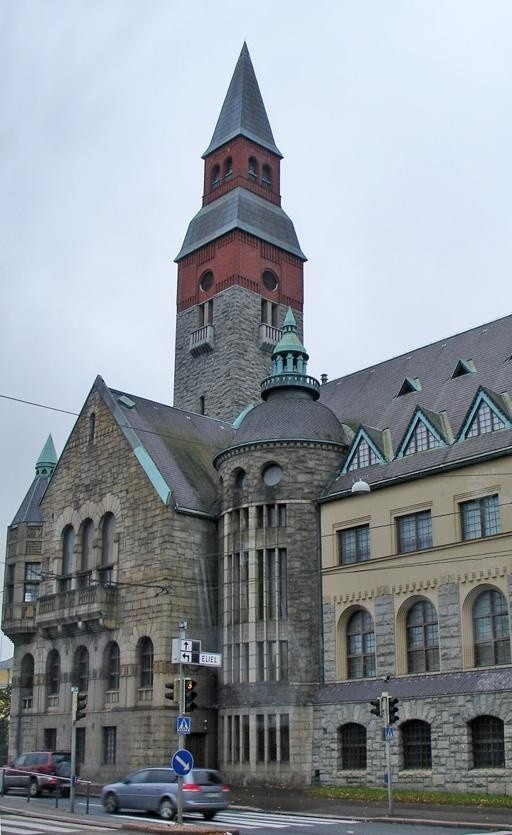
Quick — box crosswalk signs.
[176,717,190,734]
[384,727,395,741]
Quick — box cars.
[101,768,231,820]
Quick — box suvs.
[0,749,80,798]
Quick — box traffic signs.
[180,652,222,667]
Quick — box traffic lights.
[370,696,399,725]
[184,678,197,715]
[165,679,178,703]
[72,692,88,721]
